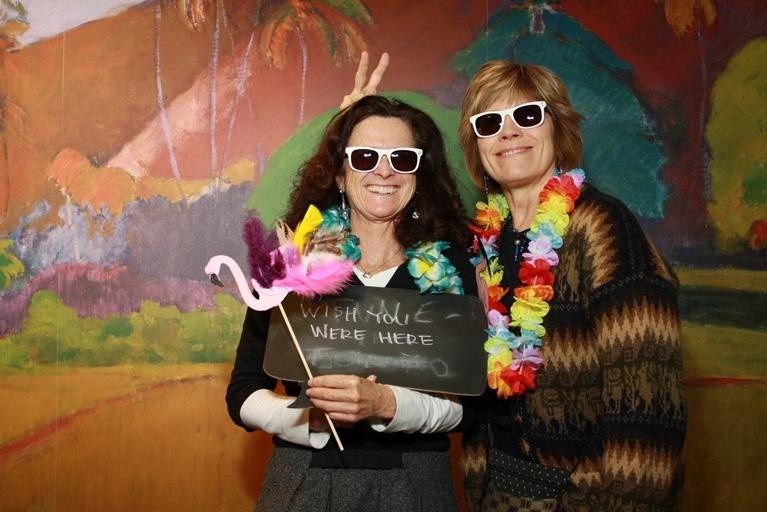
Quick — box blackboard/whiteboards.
[262,287,488,399]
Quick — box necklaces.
[355,246,402,278]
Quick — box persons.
[339,52,687,511]
[224,94,493,510]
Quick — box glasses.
[344,146,424,174]
[469,101,547,138]
